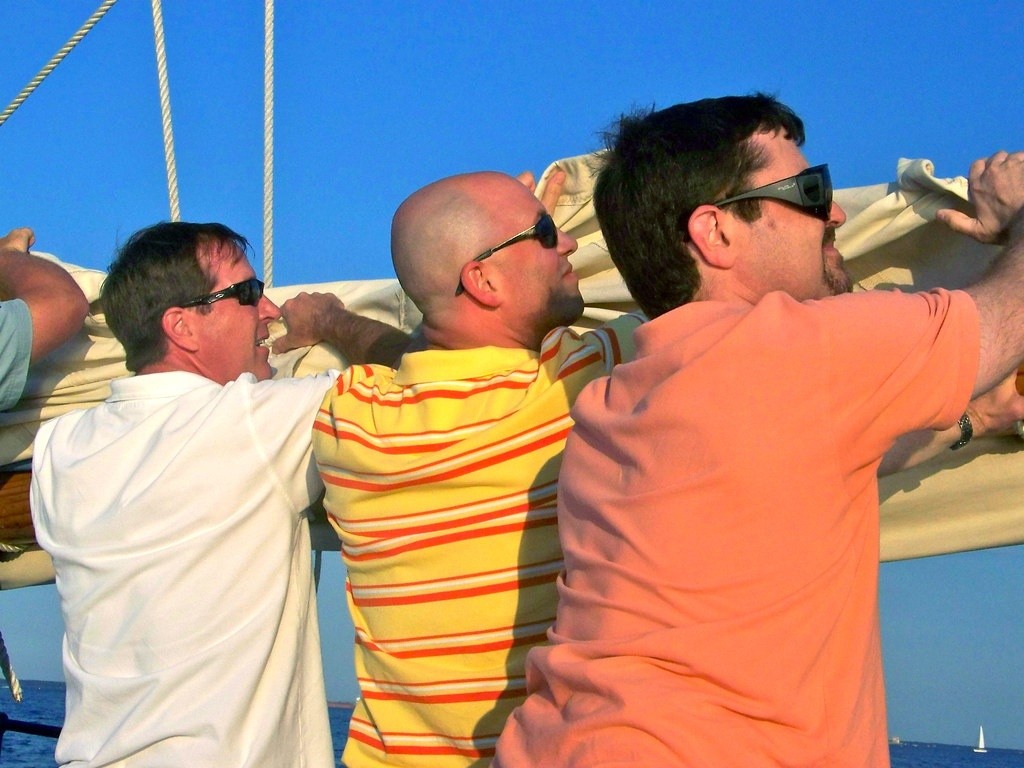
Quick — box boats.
[0,0,1024,768]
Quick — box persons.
[492,88,1023,767]
[30,219,406,767]
[312,172,653,768]
[1,228,90,412]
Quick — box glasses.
[713,162,833,221]
[455,215,558,295]
[177,279,265,308]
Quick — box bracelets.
[950,413,973,451]
